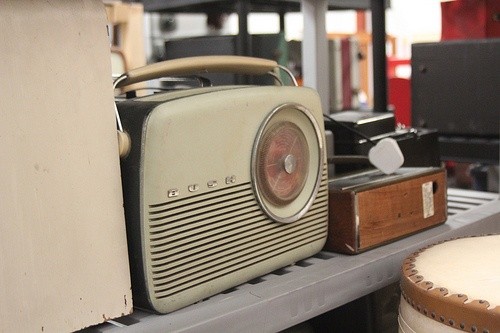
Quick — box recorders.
[113,55,329,315]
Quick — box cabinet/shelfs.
[80,0,500,333]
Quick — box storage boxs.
[0,0,134,333]
[164,32,289,88]
[326,166,449,255]
[410,38,500,140]
